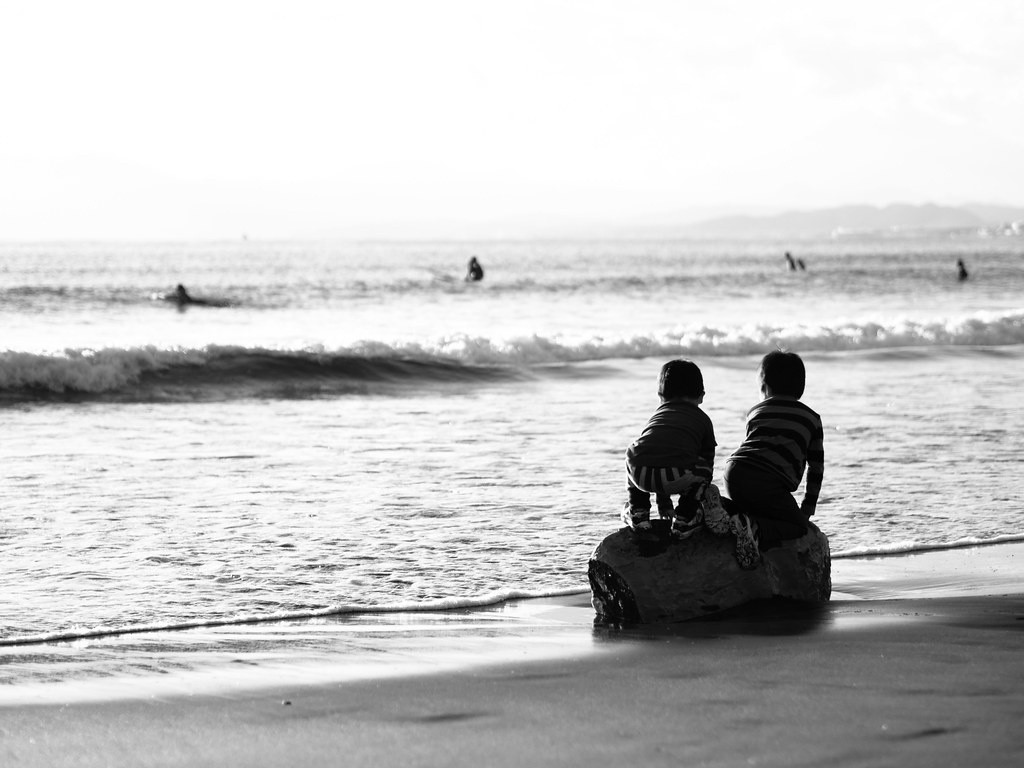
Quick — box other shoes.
[671,508,706,540]
[705,484,733,535]
[622,501,663,534]
[729,513,761,570]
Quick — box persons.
[619,359,717,541]
[174,283,191,303]
[956,258,968,281]
[467,256,484,281]
[702,345,824,570]
[784,251,805,272]
